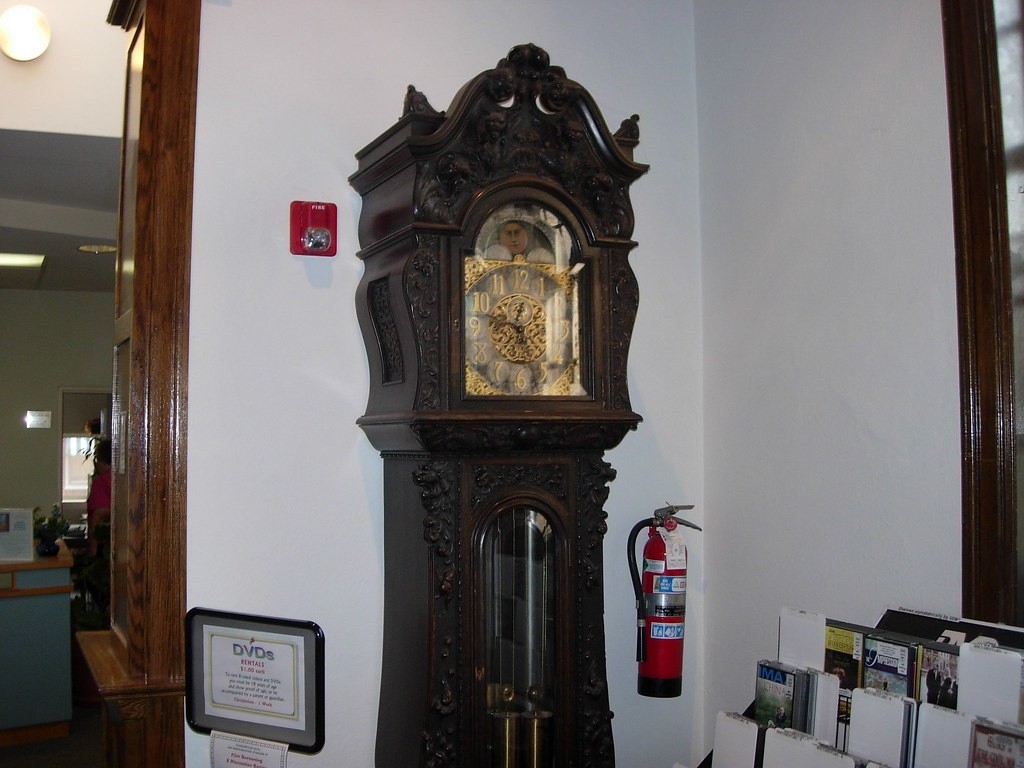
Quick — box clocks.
[349,40,651,768]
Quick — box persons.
[84,440,111,613]
[926,662,957,710]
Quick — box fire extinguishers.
[628,501,703,698]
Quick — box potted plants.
[34,503,70,556]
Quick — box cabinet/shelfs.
[73,0,199,768]
